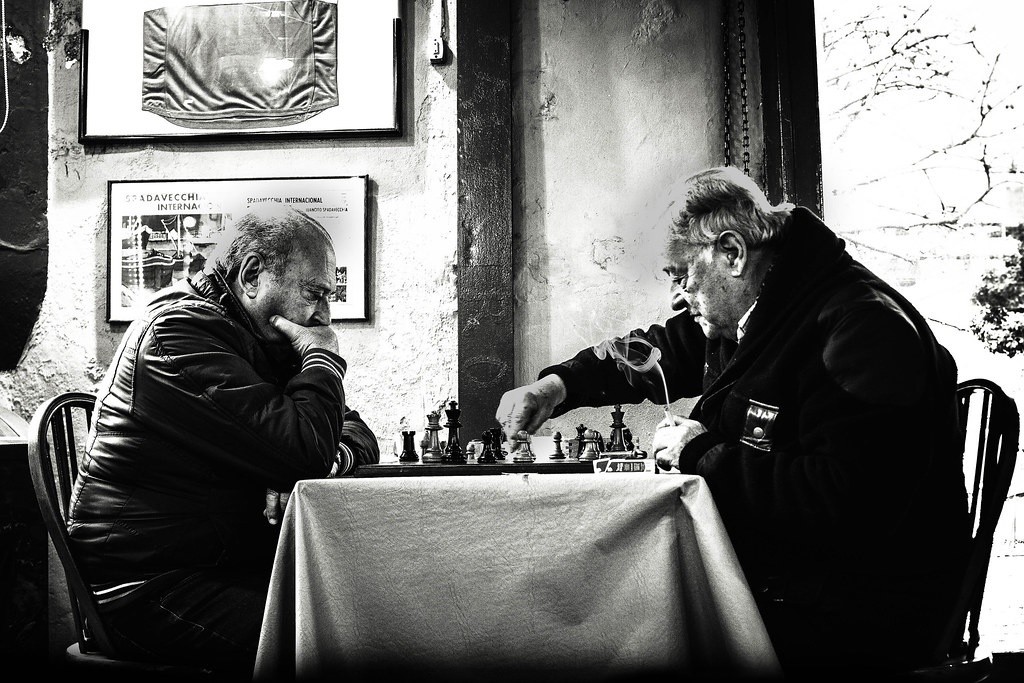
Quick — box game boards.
[354,447,597,477]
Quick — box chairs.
[904,380,1019,683]
[27,392,252,683]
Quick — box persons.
[495,165,973,683]
[66,205,379,683]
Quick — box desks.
[254,471,788,683]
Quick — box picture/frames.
[77,0,404,145]
